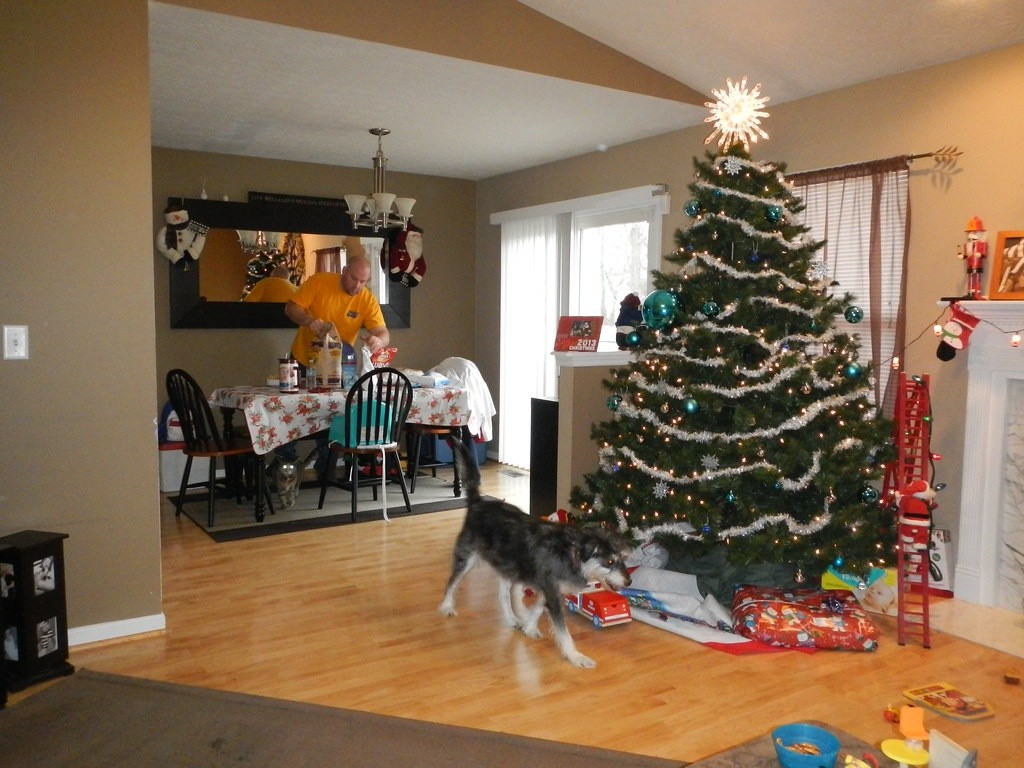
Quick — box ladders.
[881,372,932,648]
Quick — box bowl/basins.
[772,723,840,768]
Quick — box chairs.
[318,368,413,521]
[165,370,266,527]
[404,358,497,497]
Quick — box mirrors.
[167,196,411,330]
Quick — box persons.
[268,256,390,479]
[245,265,299,302]
[615,293,644,351]
[388,222,426,287]
[958,216,988,299]
[156,202,210,271]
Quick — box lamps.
[344,126,415,232]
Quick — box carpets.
[170,469,503,544]
[681,718,918,768]
[0,667,701,768]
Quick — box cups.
[279,359,301,394]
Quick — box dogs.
[436,434,633,670]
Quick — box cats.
[271,446,332,511]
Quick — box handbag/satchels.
[360,345,378,391]
[309,320,342,389]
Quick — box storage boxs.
[435,436,487,465]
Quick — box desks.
[205,382,471,524]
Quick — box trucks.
[565,580,633,629]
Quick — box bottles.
[306,360,316,390]
